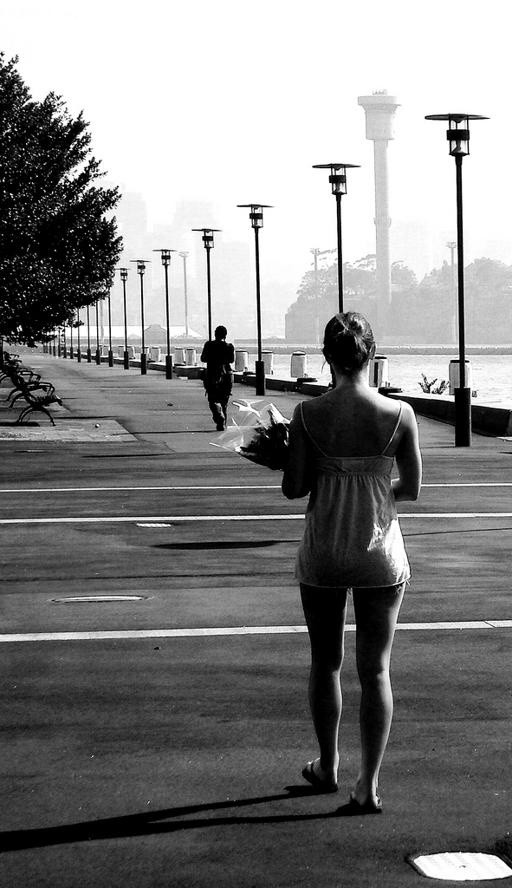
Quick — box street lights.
[191,228,222,341]
[236,204,274,396]
[425,113,491,447]
[115,268,131,369]
[153,249,177,379]
[130,260,151,374]
[312,163,362,312]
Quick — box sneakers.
[215,416,224,431]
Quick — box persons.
[201,325,235,431]
[279,312,423,815]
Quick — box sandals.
[300,760,340,793]
[350,790,384,815]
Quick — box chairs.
[0,351,63,427]
